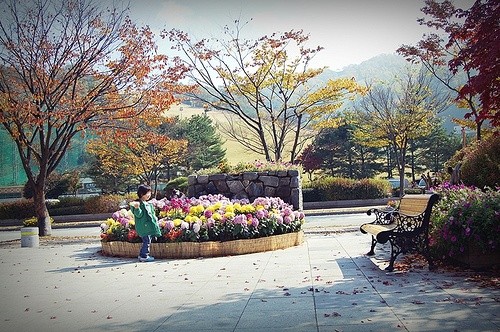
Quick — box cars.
[418,178,442,189]
[388,178,408,190]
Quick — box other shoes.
[138,255,155,262]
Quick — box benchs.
[360,193,440,271]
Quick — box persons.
[129,186,162,262]
[409,171,439,192]
